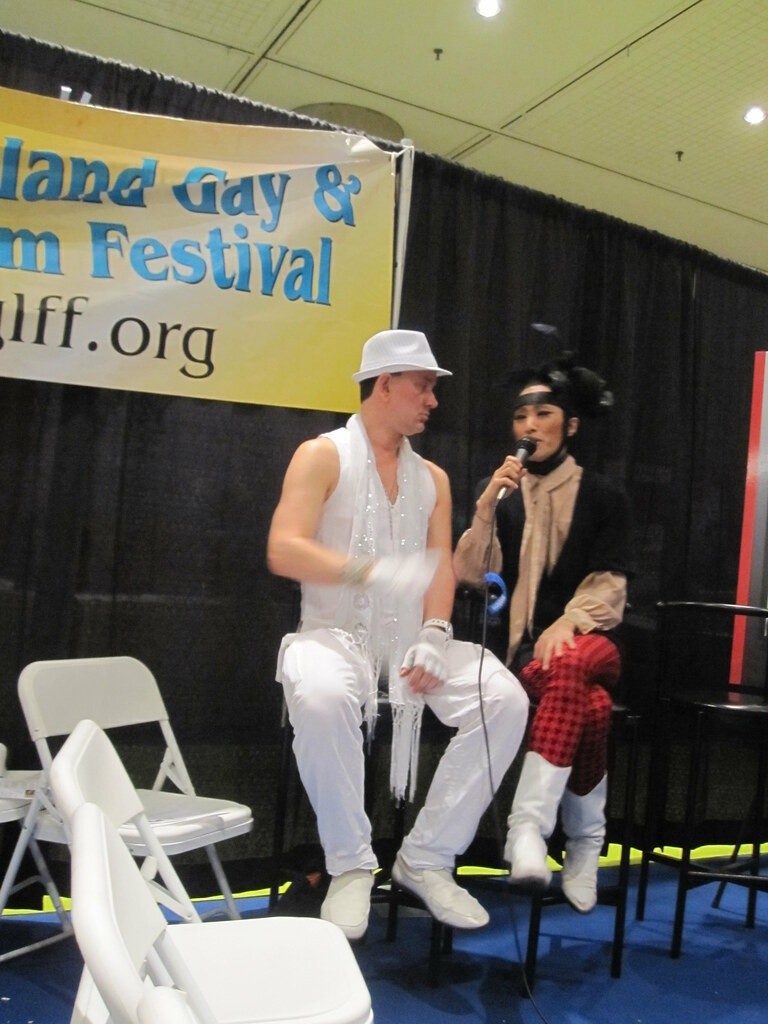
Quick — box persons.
[269,329,531,939]
[452,365,641,913]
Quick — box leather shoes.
[321,869,374,939]
[391,854,491,930]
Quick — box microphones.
[494,436,537,509]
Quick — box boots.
[502,750,573,883]
[562,769,608,914]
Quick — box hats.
[352,330,452,385]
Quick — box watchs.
[421,618,454,638]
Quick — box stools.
[265,589,767,1002]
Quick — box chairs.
[0,654,254,923]
[0,768,78,963]
[50,721,374,1023]
[68,803,222,1023]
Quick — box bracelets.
[344,558,364,587]
